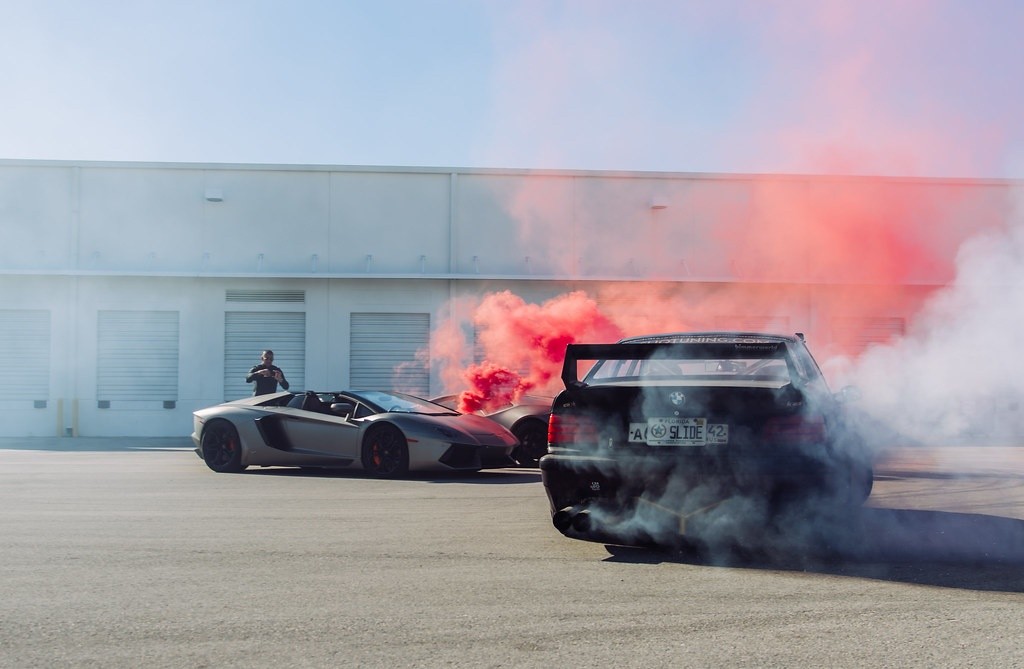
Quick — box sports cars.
[191,389,519,481]
[541,330,872,556]
[422,391,553,466]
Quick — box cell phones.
[264,372,275,378]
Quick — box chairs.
[331,404,352,416]
[644,361,681,376]
[757,365,794,377]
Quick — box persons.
[245,350,290,397]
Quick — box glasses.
[262,356,274,361]
